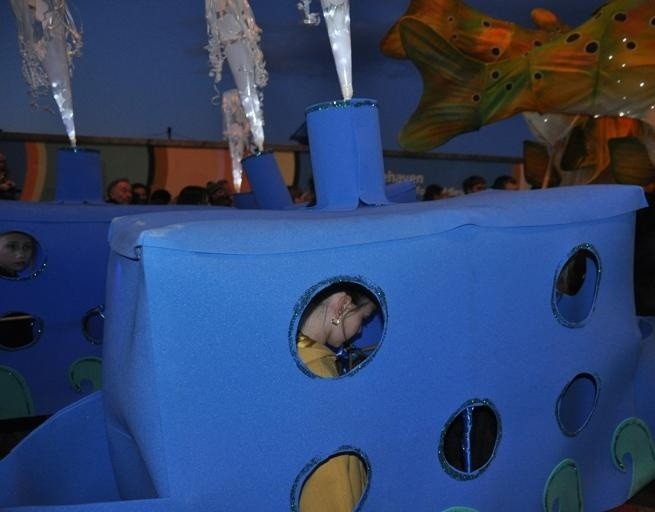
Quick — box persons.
[0,231,33,278]
[290,280,379,378]
[130,183,148,203]
[176,186,208,205]
[106,178,133,204]
[462,176,487,195]
[150,189,171,205]
[218,180,236,195]
[499,175,518,190]
[0,153,18,200]
[423,184,443,201]
[207,182,232,206]
